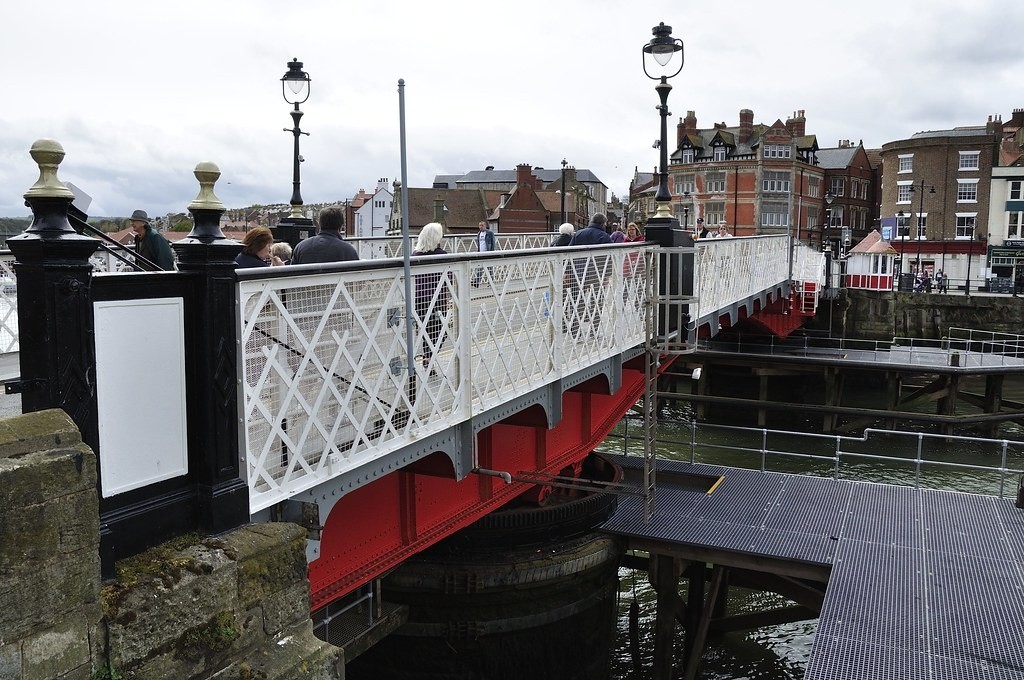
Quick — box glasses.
[697,222,703,224]
[720,228,726,230]
[628,229,636,231]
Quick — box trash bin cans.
[898,273,915,292]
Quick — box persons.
[550,223,574,332]
[565,212,615,344]
[471,219,495,288]
[235,225,273,269]
[609,231,625,243]
[924,269,928,278]
[715,224,734,289]
[128,209,174,271]
[692,218,714,292]
[290,208,360,265]
[270,242,293,266]
[412,221,453,377]
[936,269,945,283]
[926,274,932,293]
[938,272,948,293]
[623,222,646,322]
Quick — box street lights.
[965,217,979,295]
[809,223,829,246]
[642,22,684,241]
[824,188,835,256]
[276,57,316,244]
[909,179,936,274]
[680,191,692,230]
[897,209,913,275]
[560,159,568,225]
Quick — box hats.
[697,218,704,222]
[128,209,150,225]
